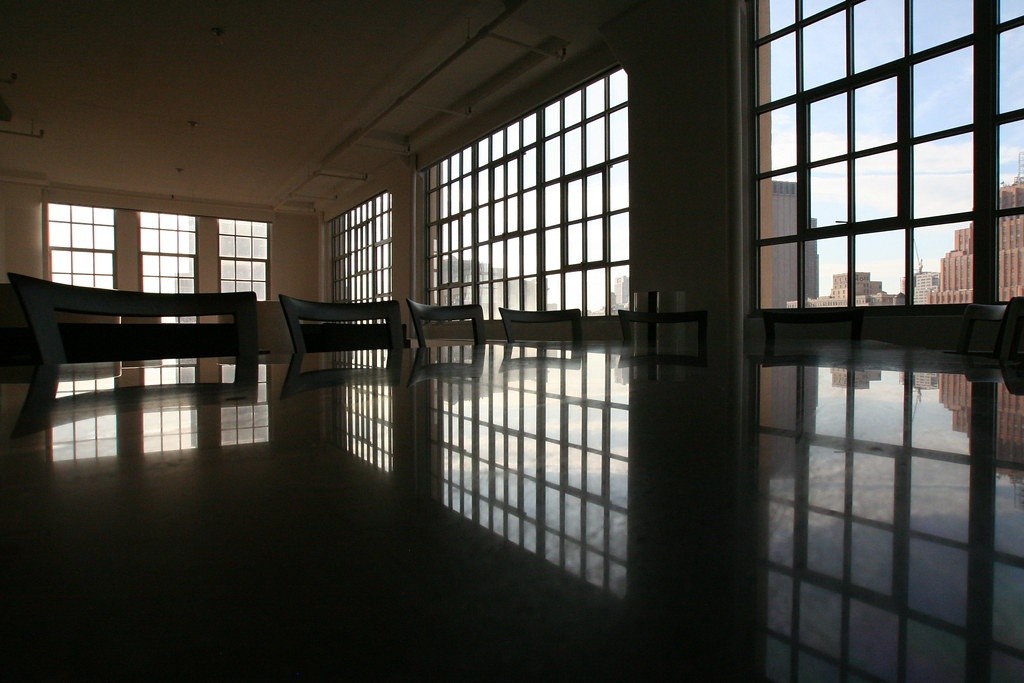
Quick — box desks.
[0,341,1024,683]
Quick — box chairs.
[7,272,259,364]
[618,309,708,345]
[278,294,405,353]
[762,309,865,341]
[956,297,1024,361]
[404,296,486,348]
[498,306,584,342]
